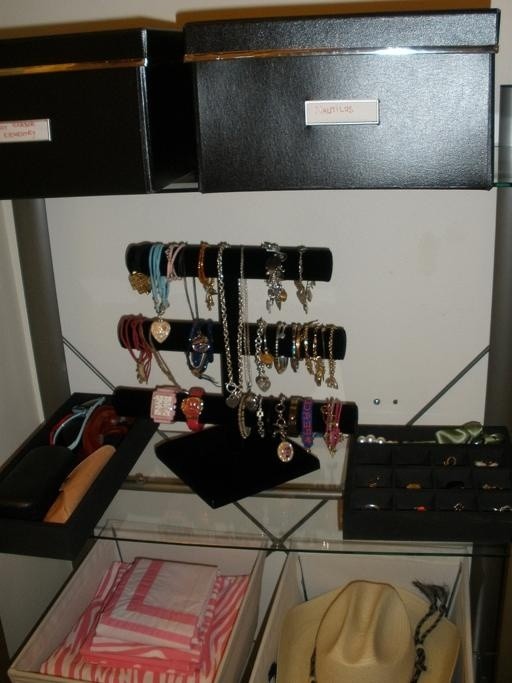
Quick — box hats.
[275,579,461,683]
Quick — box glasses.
[50,396,106,450]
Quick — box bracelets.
[253,241,349,464]
[119,239,218,391]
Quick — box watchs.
[150,387,181,425]
[181,385,210,432]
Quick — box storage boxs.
[239,537,476,682]
[0,28,197,195]
[1,520,268,681]
[181,6,501,193]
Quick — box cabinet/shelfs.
[2,0,512,683]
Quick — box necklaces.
[237,244,257,439]
[214,238,247,408]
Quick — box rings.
[454,504,466,512]
[366,477,380,488]
[406,480,421,489]
[482,482,503,490]
[489,504,511,512]
[363,503,381,511]
[443,456,456,466]
[413,505,428,512]
